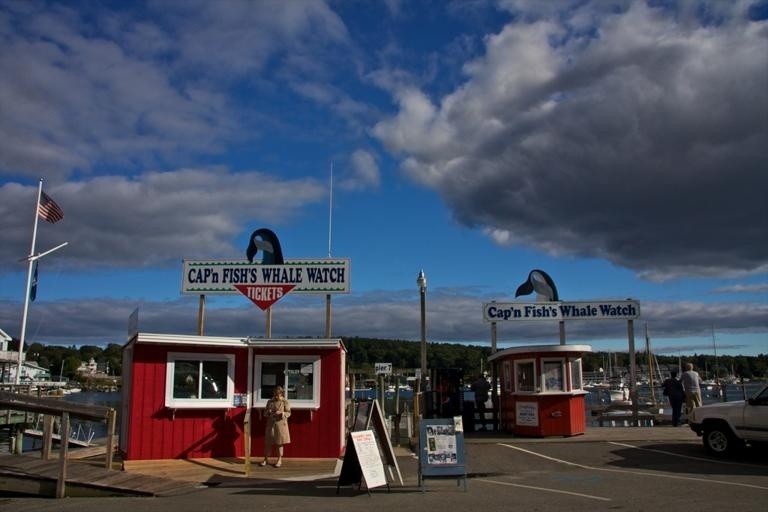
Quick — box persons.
[662,370,686,426]
[679,362,703,424]
[260,385,292,467]
[472,375,491,431]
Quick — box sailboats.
[582,321,751,415]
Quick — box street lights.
[416,267,428,394]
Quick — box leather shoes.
[275,461,282,467]
[260,461,267,466]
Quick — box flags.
[30,261,39,301]
[38,191,64,224]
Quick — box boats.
[345,379,414,400]
